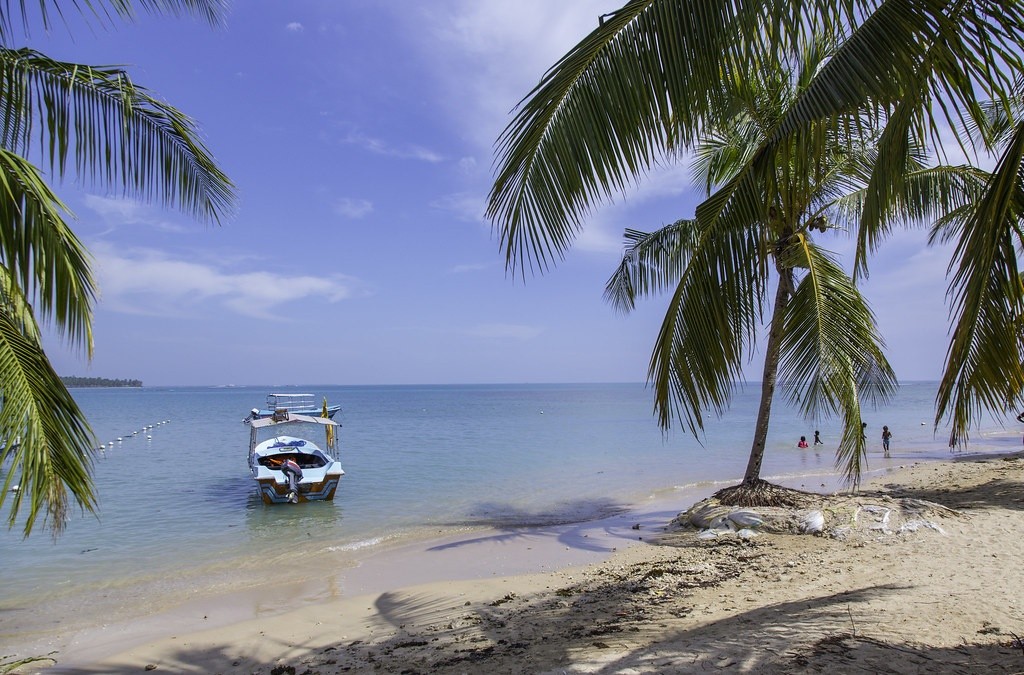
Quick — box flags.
[322,399,334,447]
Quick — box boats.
[241,407,346,505]
[241,394,342,424]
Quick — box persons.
[269,454,319,466]
[862,422,867,440]
[798,436,808,447]
[814,430,823,445]
[882,425,892,451]
[1017,412,1024,443]
